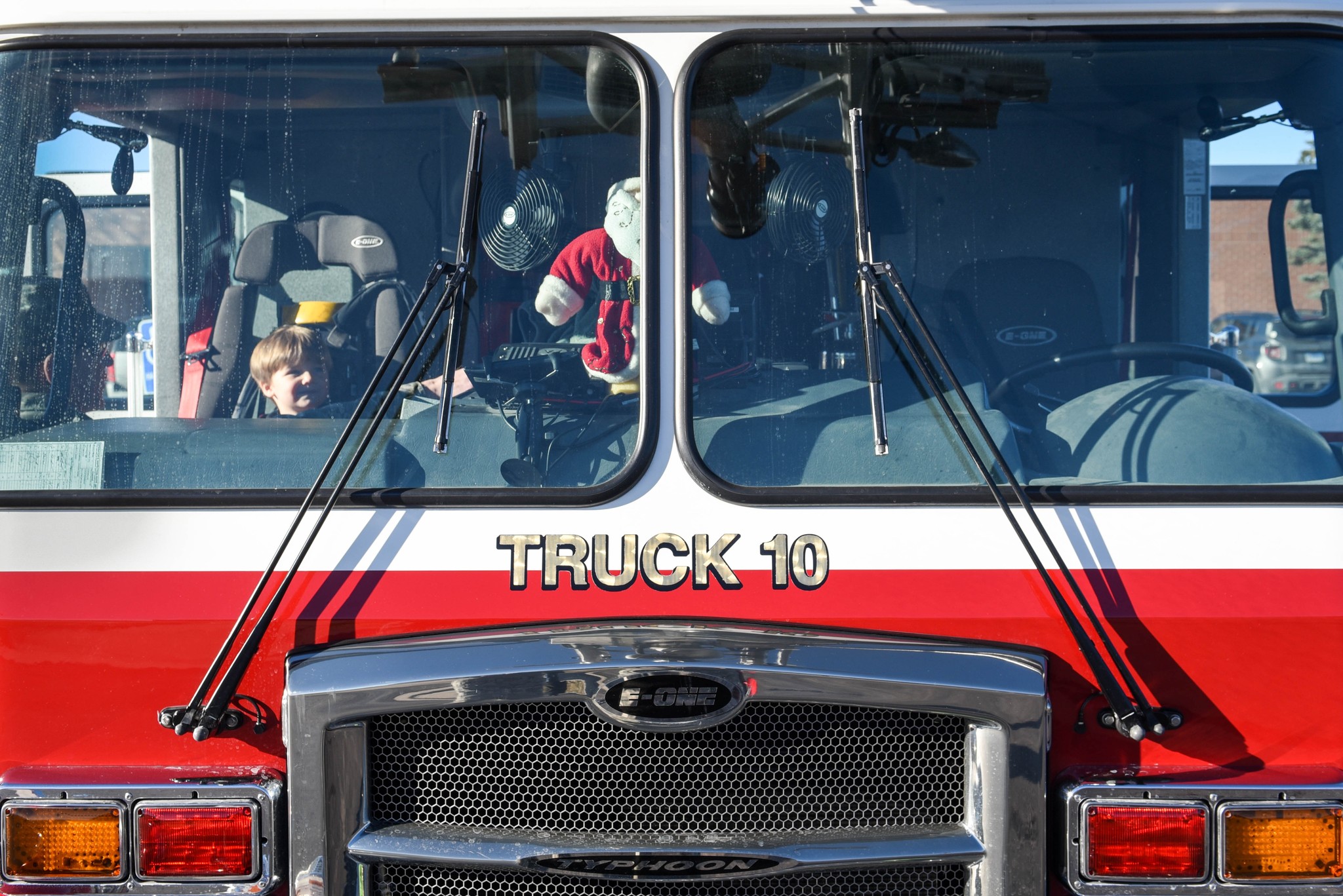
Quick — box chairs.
[191,214,409,420]
[948,252,1127,421]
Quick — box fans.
[476,158,571,276]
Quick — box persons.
[9,275,474,433]
[250,322,332,419]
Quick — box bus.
[21,171,250,418]
[3,1,1341,896]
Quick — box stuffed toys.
[533,177,731,386]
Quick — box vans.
[1206,309,1335,399]
